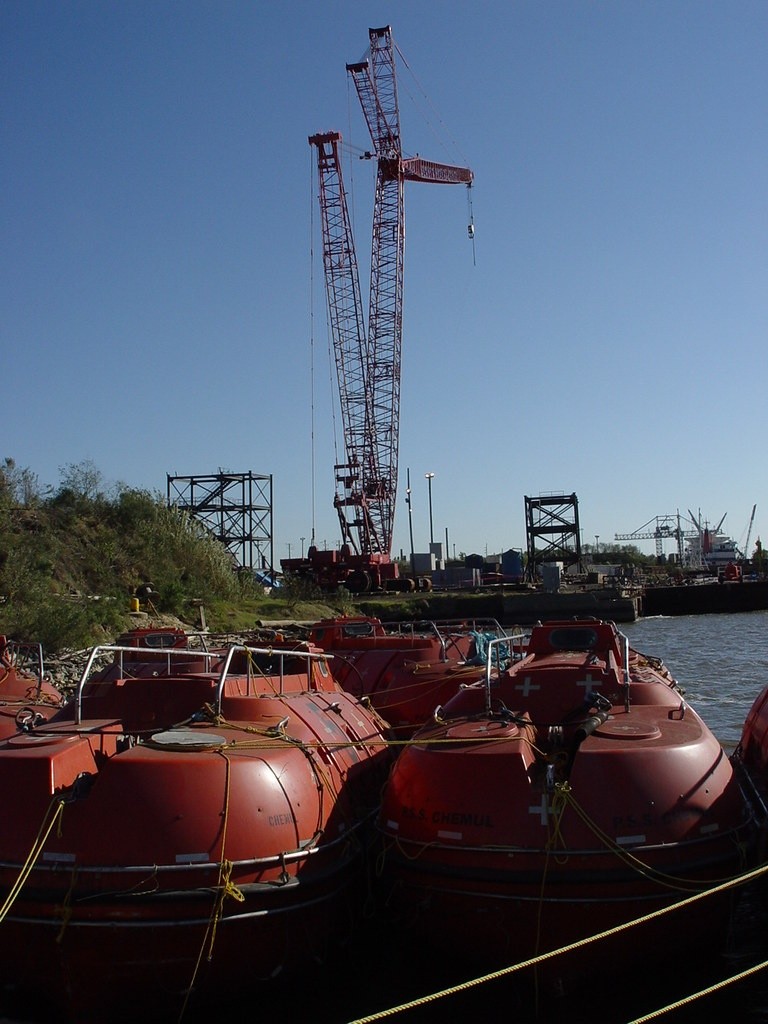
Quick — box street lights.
[423,472,436,543]
[405,468,416,578]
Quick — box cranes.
[281,24,476,594]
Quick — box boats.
[1,622,766,1023]
